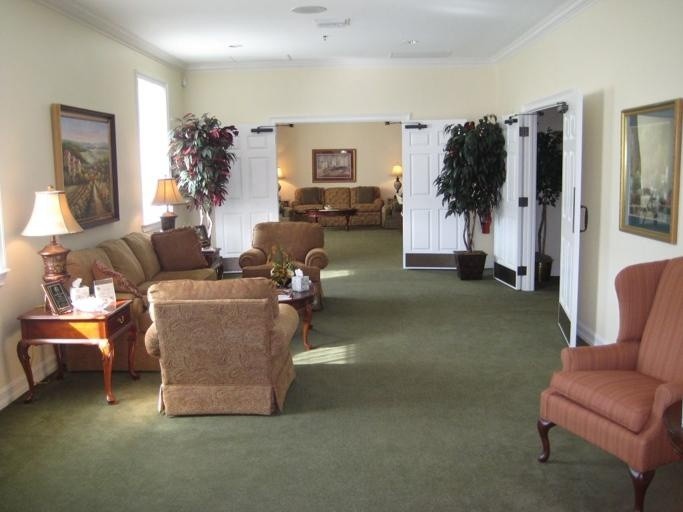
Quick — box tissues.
[69,278,89,300]
[292,269,309,291]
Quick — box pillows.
[90,256,148,309]
[151,225,208,269]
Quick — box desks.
[15,297,143,405]
[201,248,224,280]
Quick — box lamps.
[151,177,185,231]
[21,184,84,306]
[390,166,403,193]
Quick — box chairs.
[381,195,402,229]
[140,275,301,418]
[536,255,683,512]
[280,200,293,222]
[238,221,328,313]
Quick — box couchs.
[63,229,226,373]
[291,185,384,227]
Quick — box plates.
[71,297,114,312]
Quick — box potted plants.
[432,112,507,280]
[536,125,562,288]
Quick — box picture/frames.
[312,149,356,183]
[620,98,683,245]
[40,280,73,315]
[51,102,121,230]
[191,224,211,247]
